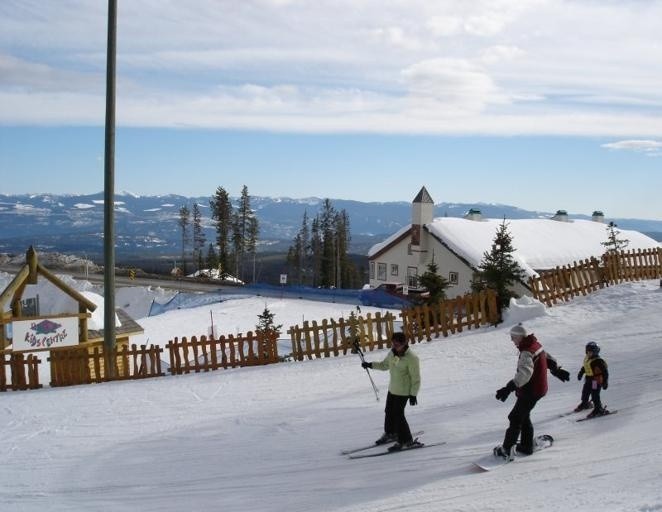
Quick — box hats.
[584,342,599,358]
[392,332,407,344]
[510,323,528,337]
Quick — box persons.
[577,341,610,417]
[361,332,421,450]
[494,323,568,463]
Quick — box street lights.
[328,227,340,288]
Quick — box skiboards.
[339,431,446,460]
[559,401,619,422]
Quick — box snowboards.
[472,435,554,471]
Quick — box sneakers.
[492,442,533,458]
[578,400,605,417]
[378,431,415,452]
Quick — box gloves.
[556,368,570,382]
[578,371,583,381]
[496,387,509,403]
[600,381,608,390]
[407,394,418,407]
[362,362,374,370]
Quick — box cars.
[358,283,409,306]
